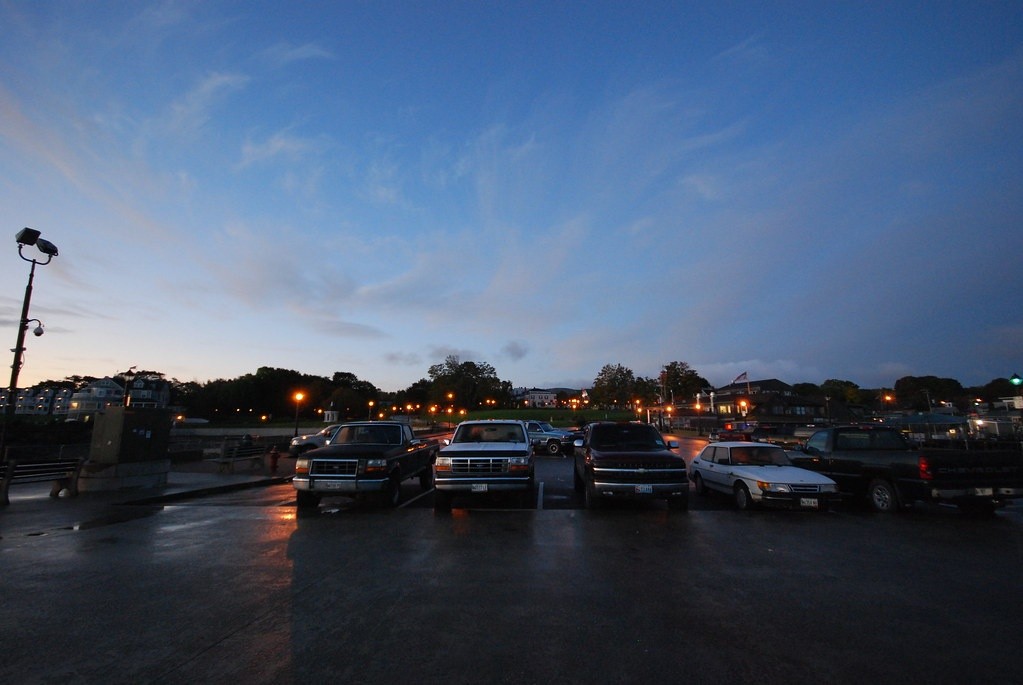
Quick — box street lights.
[393,407,396,421]
[667,406,673,429]
[886,396,891,415]
[695,404,701,436]
[368,402,373,421]
[447,393,453,432]
[294,393,304,437]
[1,228,59,507]
[407,405,411,422]
[824,393,832,428]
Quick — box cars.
[688,441,839,510]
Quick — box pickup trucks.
[292,421,442,508]
[785,425,1023,512]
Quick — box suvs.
[432,417,535,494]
[525,420,574,456]
[289,424,341,458]
[573,420,690,510]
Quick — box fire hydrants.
[269,446,281,473]
